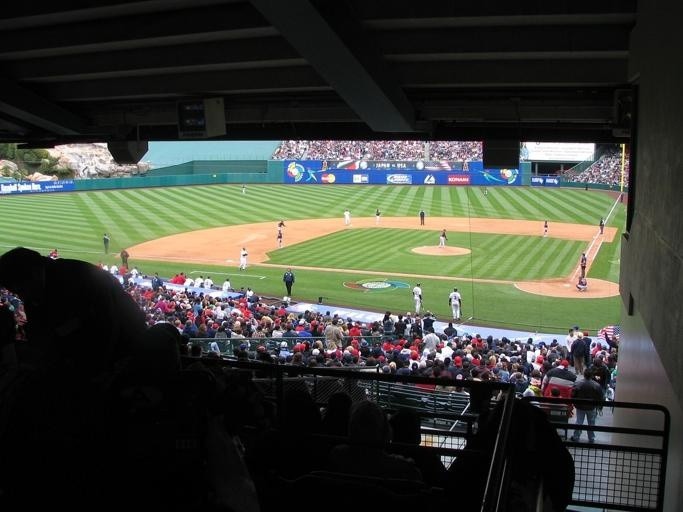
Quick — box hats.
[577,331,588,339]
[480,359,485,365]
[493,368,499,374]
[382,361,396,374]
[455,356,462,366]
[561,359,568,366]
[361,340,369,347]
[537,355,543,363]
[293,344,306,351]
[397,338,421,359]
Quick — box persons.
[277,230,283,248]
[484,186,488,195]
[278,220,286,231]
[544,221,548,238]
[376,209,381,224]
[568,151,629,190]
[439,229,448,247]
[102,233,110,254]
[271,140,483,161]
[344,209,352,225]
[599,217,604,234]
[419,210,425,226]
[0,247,622,511]
[242,184,246,195]
